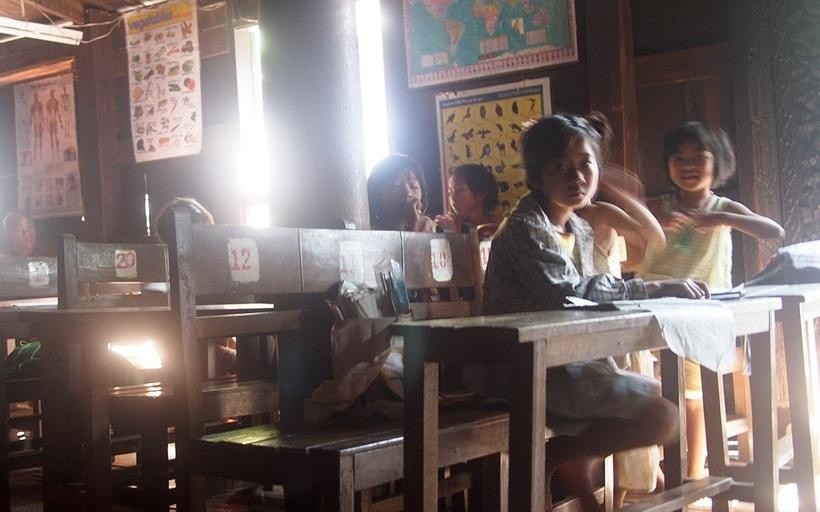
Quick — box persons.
[367,152,438,303]
[632,121,786,479]
[154,196,274,375]
[574,110,667,511]
[435,163,503,301]
[463,111,680,512]
[2,210,36,257]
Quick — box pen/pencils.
[379,271,404,313]
[711,291,742,296]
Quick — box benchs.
[57,232,171,307]
[110,210,492,511]
[3,246,55,449]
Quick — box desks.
[739,287,820,511]
[392,294,783,512]
[21,299,275,507]
